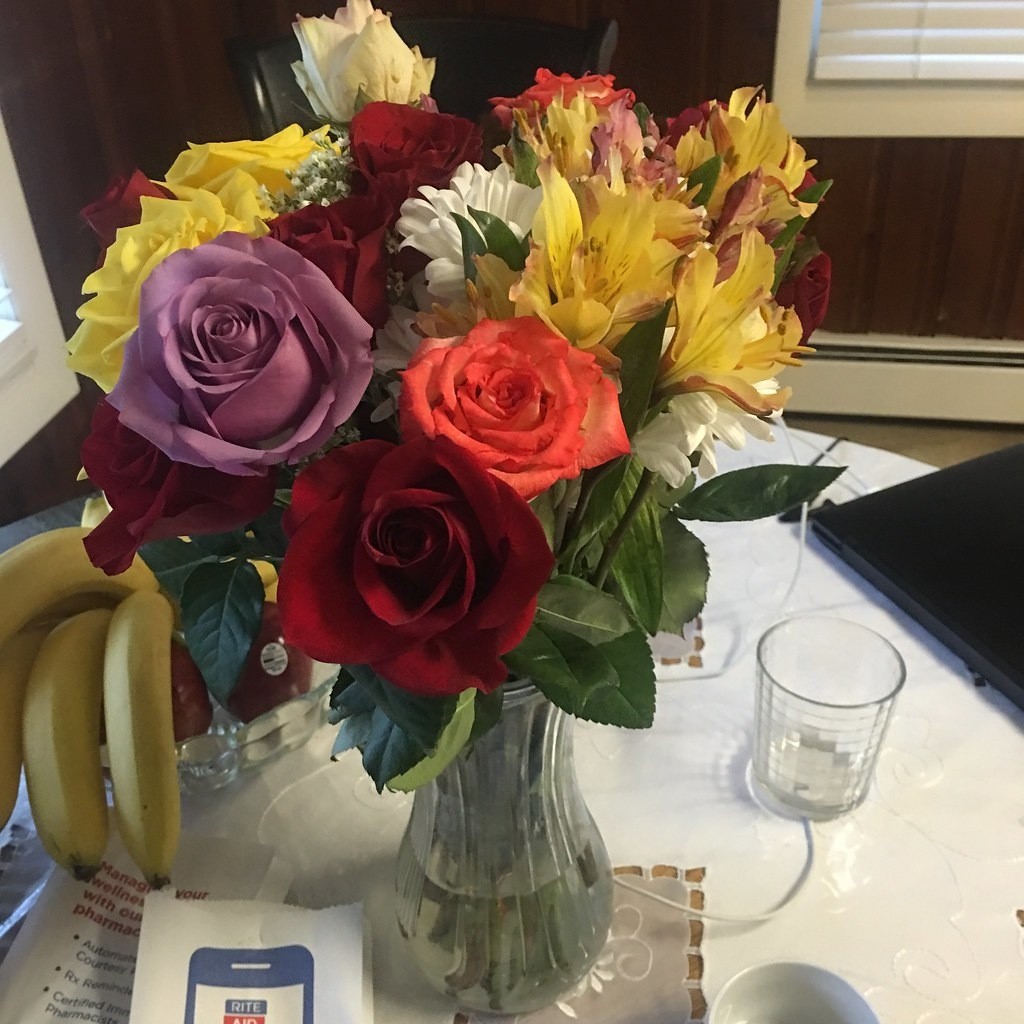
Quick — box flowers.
[60,0,848,1007]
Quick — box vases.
[391,676,615,1016]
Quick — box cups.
[751,617,905,816]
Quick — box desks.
[0,428,1024,1024]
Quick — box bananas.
[0,526,278,887]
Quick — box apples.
[170,597,311,738]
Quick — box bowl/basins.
[706,962,883,1024]
